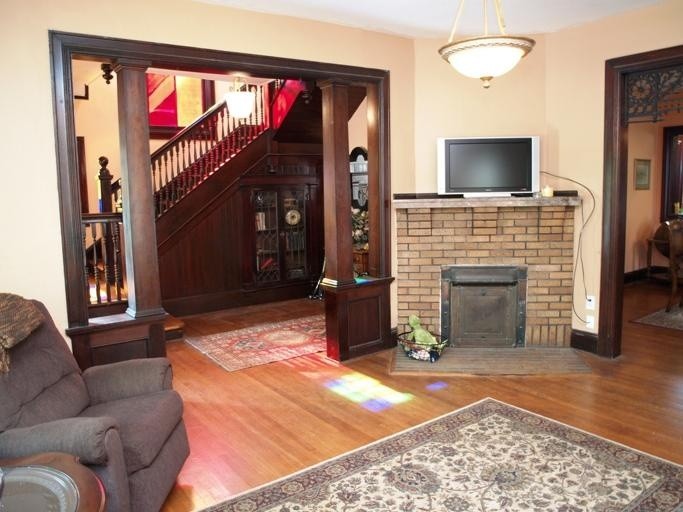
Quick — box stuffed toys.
[407,313,448,349]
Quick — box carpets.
[389,345,592,375]
[197,396,683,512]
[184,314,329,373]
[627,304,683,332]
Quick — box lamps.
[222,77,255,120]
[436,0,537,91]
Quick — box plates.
[285,209,301,225]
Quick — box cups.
[540,184,553,196]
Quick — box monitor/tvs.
[436,135,540,198]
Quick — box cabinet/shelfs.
[239,177,320,292]
[352,251,368,273]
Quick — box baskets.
[397,331,449,361]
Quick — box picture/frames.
[145,72,217,142]
[632,157,651,190]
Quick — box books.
[259,257,272,270]
[257,212,265,229]
[256,256,259,271]
[254,213,261,231]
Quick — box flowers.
[350,205,369,250]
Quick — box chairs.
[0,300,190,512]
[663,218,683,313]
